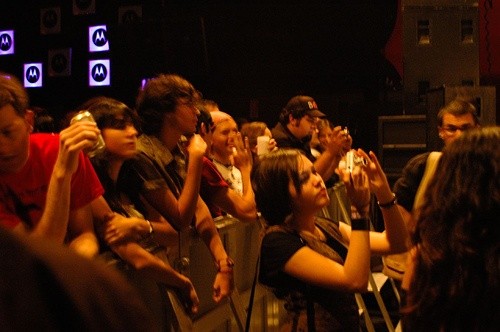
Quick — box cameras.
[347,151,367,173]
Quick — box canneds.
[70,111,106,157]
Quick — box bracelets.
[351,219,371,230]
[219,270,235,273]
[215,257,234,272]
[351,202,369,212]
[147,220,154,236]
[377,193,396,208]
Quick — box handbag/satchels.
[383,151,443,280]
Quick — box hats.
[286,96,325,117]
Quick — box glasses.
[182,100,196,109]
[439,127,475,137]
[109,118,139,131]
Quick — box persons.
[0,74,500,332]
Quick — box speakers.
[378,86,496,173]
